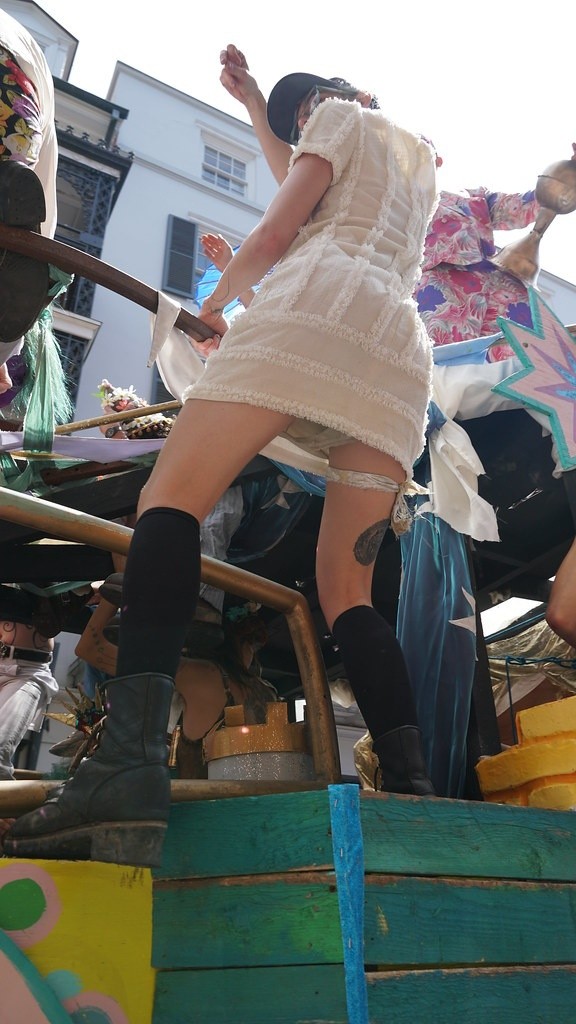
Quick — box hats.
[266,72,380,145]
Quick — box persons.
[0,9,576,868]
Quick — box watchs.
[104,425,122,438]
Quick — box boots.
[371,726,437,797]
[2,672,176,867]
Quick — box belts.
[1,647,52,662]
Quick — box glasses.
[294,84,355,127]
